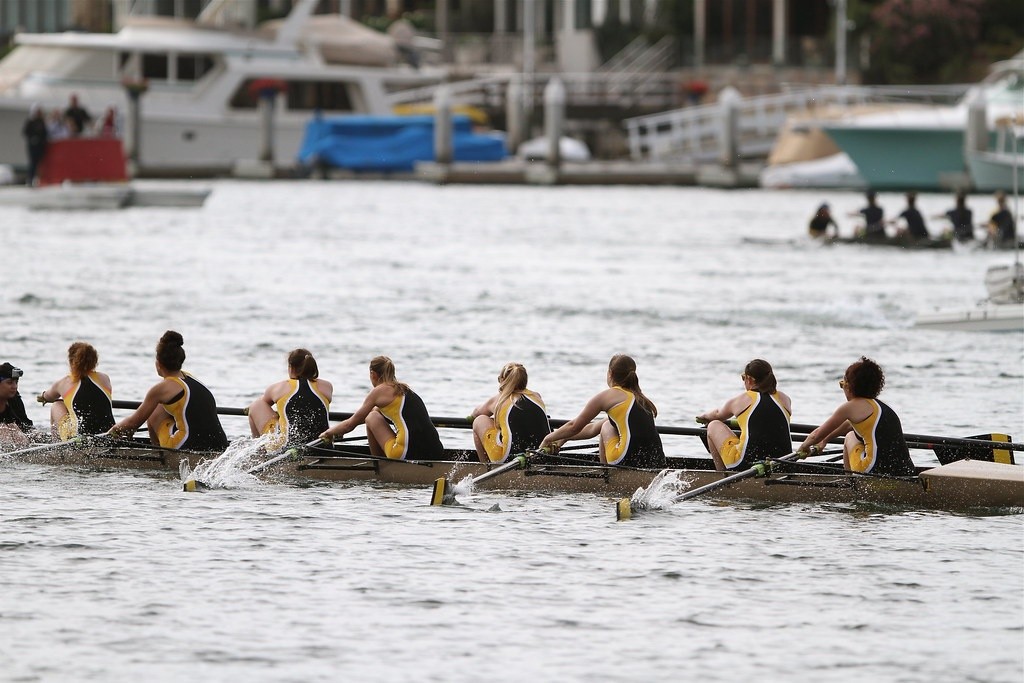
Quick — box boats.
[740,231,1023,250]
[25,180,209,208]
[0,29,407,175]
[0,423,1022,513]
[759,108,1023,195]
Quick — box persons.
[93,330,229,454]
[809,189,1014,238]
[539,354,666,469]
[0,362,34,435]
[20,93,118,186]
[471,363,554,464]
[696,358,791,472]
[318,356,443,460]
[796,356,914,476]
[248,349,334,456]
[36,342,115,445]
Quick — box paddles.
[37,396,554,432]
[183,436,330,492]
[616,445,817,523]
[0,430,133,459]
[466,417,1015,465]
[244,407,740,453]
[430,443,557,506]
[695,417,1024,451]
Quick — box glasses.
[839,380,848,388]
[741,374,755,382]
[498,376,504,383]
